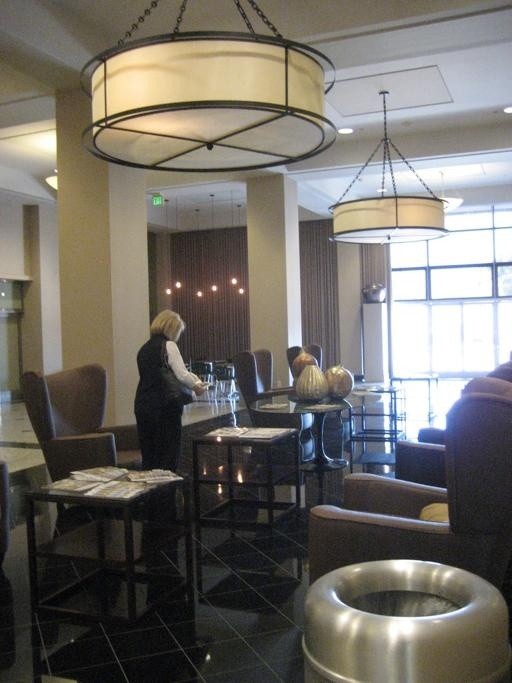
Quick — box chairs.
[186,359,239,399]
[20,363,144,518]
[232,344,367,464]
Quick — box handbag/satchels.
[161,340,196,405]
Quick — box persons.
[134,310,211,474]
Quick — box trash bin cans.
[302,558,512,683]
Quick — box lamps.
[327,90,450,244]
[81,0,338,172]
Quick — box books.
[189,371,212,390]
[39,464,185,503]
[205,423,295,441]
